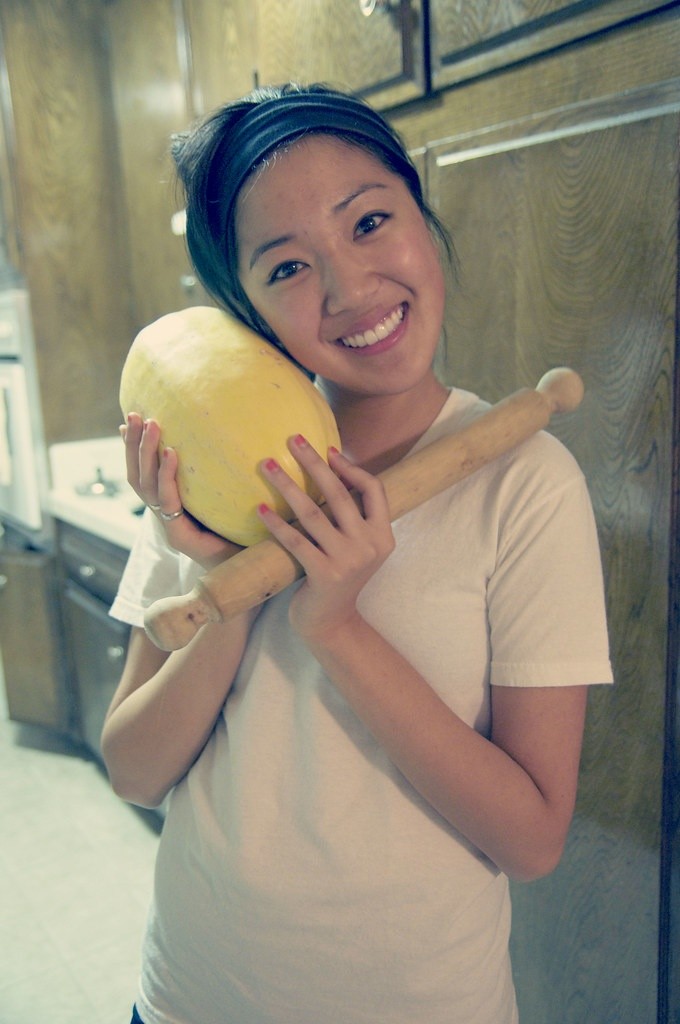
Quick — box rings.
[161,508,188,521]
[150,505,160,510]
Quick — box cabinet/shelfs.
[0,527,132,761]
[179,1,680,1017]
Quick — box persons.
[101,80,616,1024]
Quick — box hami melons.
[117,304,344,546]
